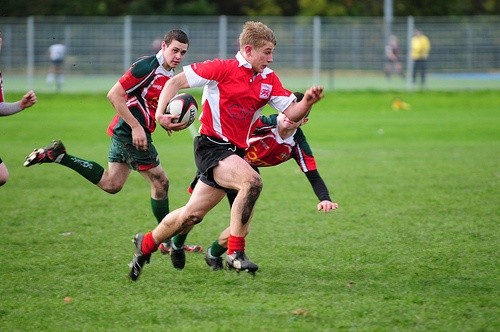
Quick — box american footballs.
[165,92,198,131]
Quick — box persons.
[24,29,203,254]
[382,34,405,80]
[410,26,431,86]
[126,20,324,281]
[171,91,339,271]
[47,37,67,93]
[0,29,37,187]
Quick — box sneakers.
[170,236,185,270]
[24,140,64,167]
[160,242,203,255]
[227,251,258,273]
[128,233,151,281]
[205,248,223,271]
